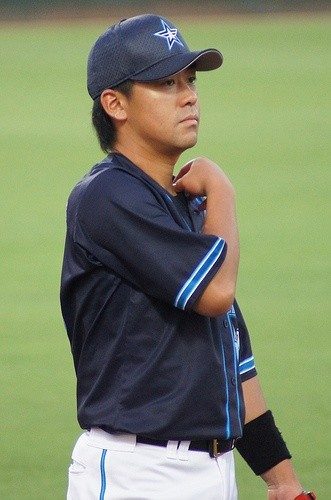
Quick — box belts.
[95,426,236,460]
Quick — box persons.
[59,14,319,500]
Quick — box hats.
[87,14,222,99]
